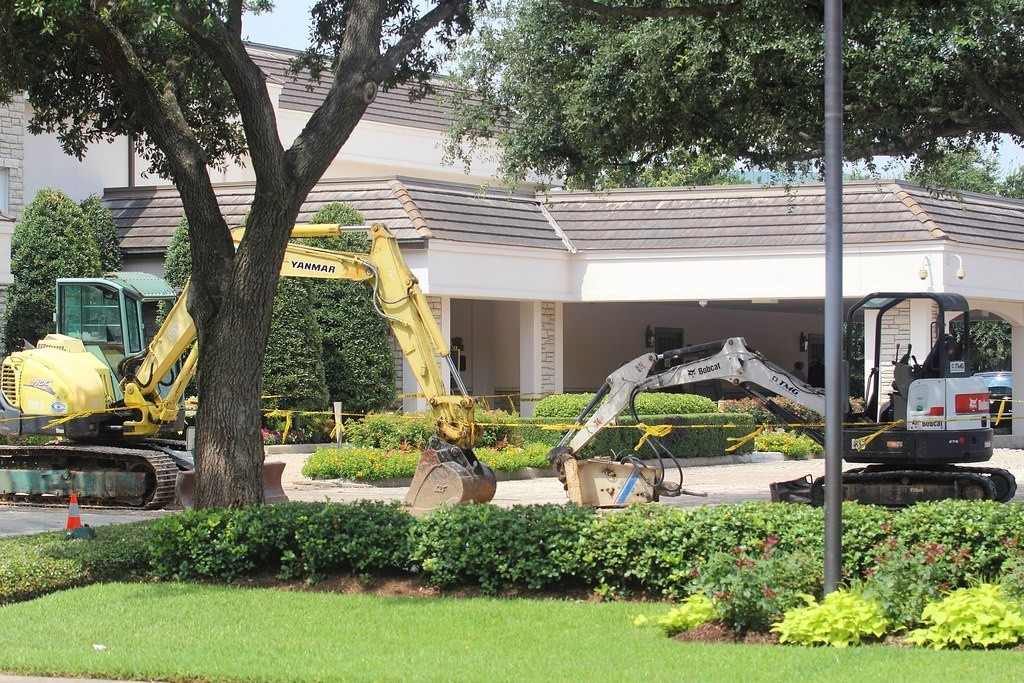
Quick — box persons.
[807,356,825,388]
[790,361,806,383]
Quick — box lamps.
[920,256,930,280]
[948,254,966,280]
[799,333,809,351]
[645,325,655,348]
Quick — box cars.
[970,371,1012,422]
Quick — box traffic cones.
[65,493,84,531]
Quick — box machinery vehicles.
[534,291,1017,514]
[1,221,499,518]
